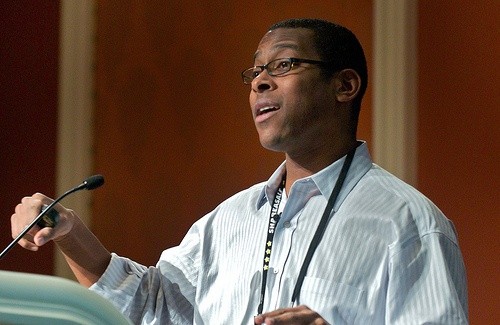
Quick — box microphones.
[273,214,280,222]
[0,175,105,261]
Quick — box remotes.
[37,205,59,228]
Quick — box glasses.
[240,56,339,85]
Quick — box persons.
[8,18,471,324]
